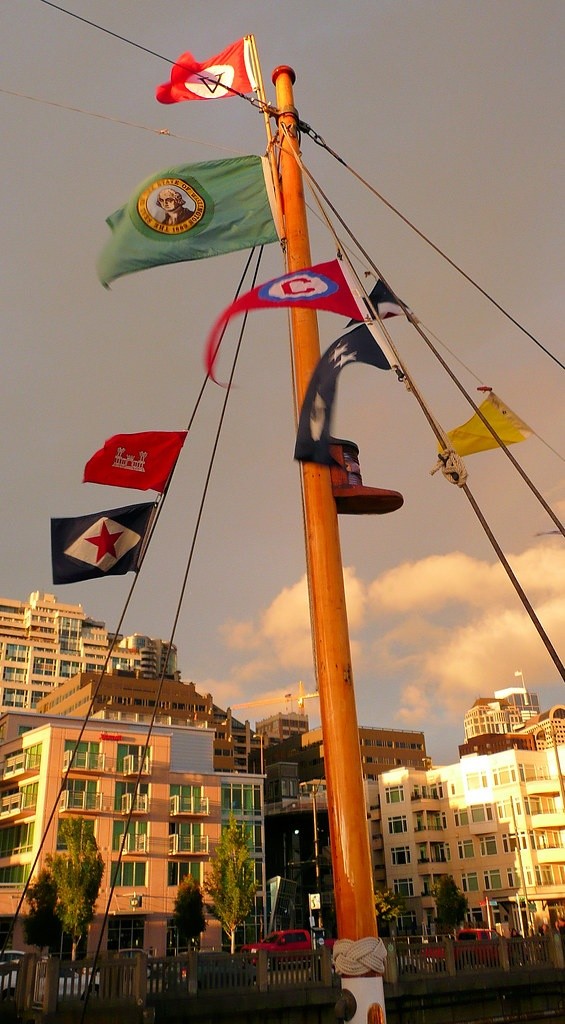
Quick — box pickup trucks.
[420,927,528,969]
[241,929,337,971]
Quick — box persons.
[508,918,565,964]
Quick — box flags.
[81,430,188,493]
[480,897,488,906]
[156,37,257,105]
[437,392,535,458]
[94,155,284,290]
[50,500,157,586]
[293,323,398,467]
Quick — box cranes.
[230,679,320,716]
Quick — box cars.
[0,948,257,1001]
